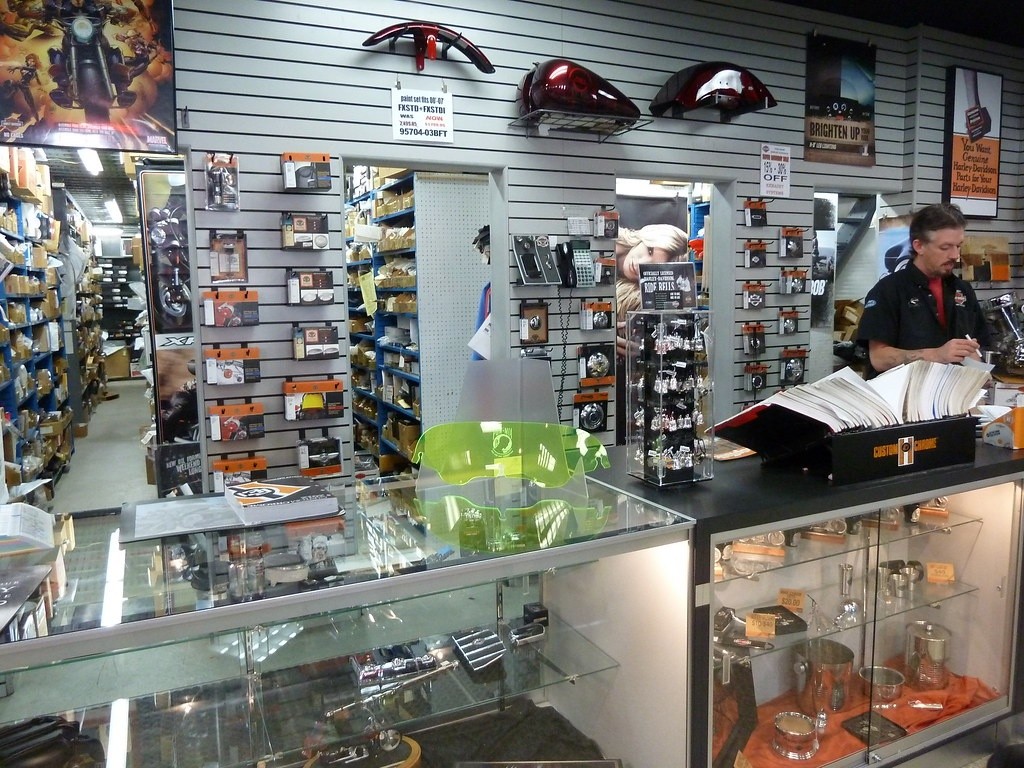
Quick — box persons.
[617,224,688,356]
[856,202,990,373]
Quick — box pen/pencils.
[965,334,986,364]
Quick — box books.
[706,360,998,464]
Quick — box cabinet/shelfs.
[94,255,154,363]
[344,172,490,534]
[0,173,74,505]
[51,182,106,427]
[542,439,1024,768]
[685,183,710,438]
[0,455,697,768]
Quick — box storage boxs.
[131,238,144,271]
[1,502,74,698]
[49,322,59,352]
[384,316,418,345]
[18,410,28,438]
[0,146,52,215]
[371,380,376,393]
[377,191,396,199]
[203,291,259,327]
[104,347,132,379]
[281,152,331,192]
[130,363,145,378]
[293,327,339,361]
[834,297,866,343]
[354,463,380,481]
[523,602,549,626]
[83,223,89,242]
[25,243,47,267]
[213,456,267,493]
[55,435,61,447]
[73,423,88,436]
[209,402,265,441]
[36,369,49,395]
[297,437,342,476]
[283,379,344,421]
[5,274,39,294]
[761,417,981,487]
[39,422,63,436]
[382,369,421,415]
[398,421,420,454]
[1,202,18,232]
[284,517,345,541]
[204,348,261,386]
[3,435,16,462]
[33,327,50,353]
[392,354,419,374]
[124,152,185,178]
[353,165,415,199]
[350,318,368,333]
[281,214,330,252]
[372,200,377,218]
[286,271,334,306]
[983,380,1024,407]
[47,289,55,317]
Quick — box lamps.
[103,193,123,223]
